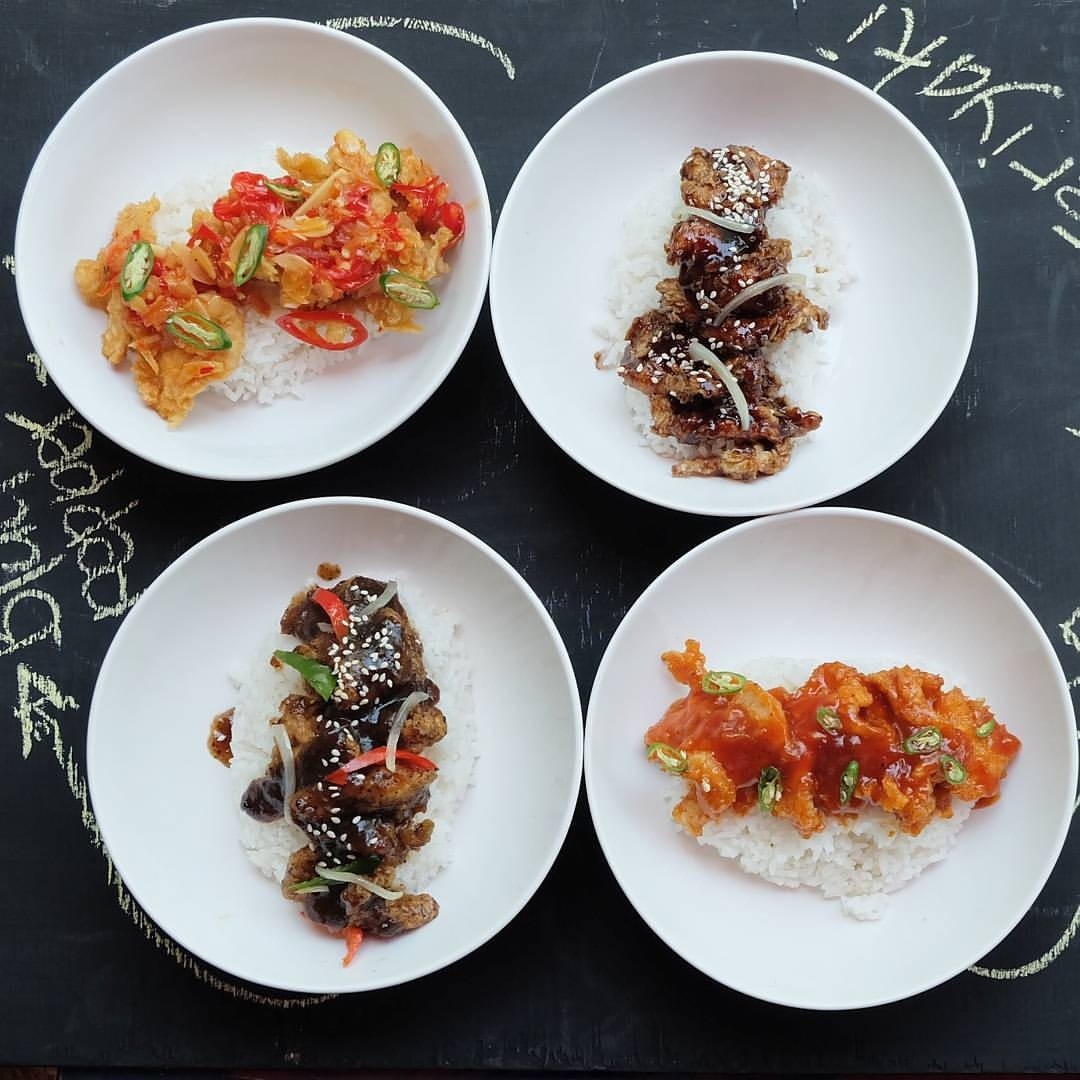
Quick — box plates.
[86,495,583,996]
[581,507,1077,1011]
[16,19,491,482]
[491,46,980,515]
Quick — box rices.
[644,639,1022,921]
[228,574,483,964]
[154,128,383,427]
[591,146,864,481]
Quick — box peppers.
[119,140,468,352]
